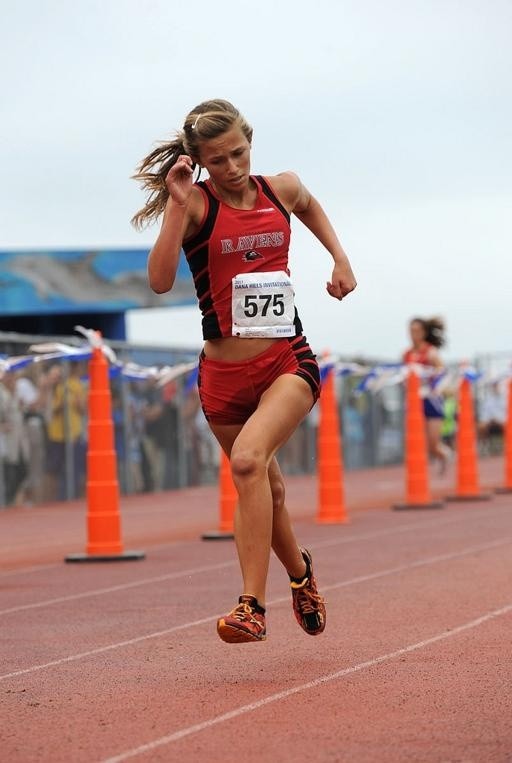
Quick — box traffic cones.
[200,447,240,540]
[68,332,145,564]
[444,379,490,501]
[493,380,512,494]
[392,373,446,509]
[308,359,350,523]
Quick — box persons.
[402,318,448,475]
[0,353,222,505]
[133,98,357,642]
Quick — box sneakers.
[217,594,266,643]
[439,447,453,475]
[290,548,328,635]
